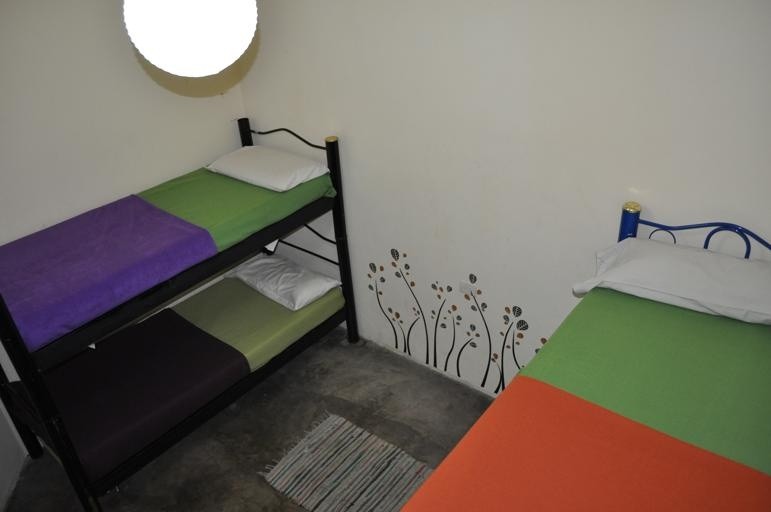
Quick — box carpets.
[255,408,436,512]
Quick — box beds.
[399,201,771,511]
[1,118,361,512]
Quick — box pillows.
[572,237,771,325]
[223,253,343,311]
[203,144,331,193]
